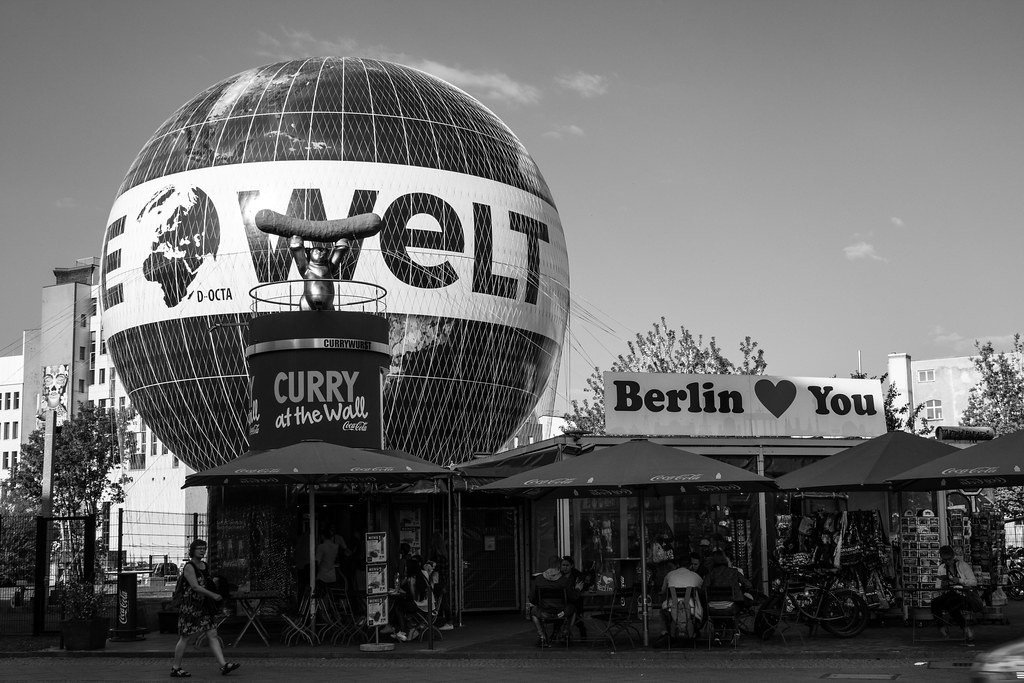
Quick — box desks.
[582,590,620,618]
[230,590,279,648]
[349,587,406,646]
[889,587,986,646]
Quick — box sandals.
[220,662,240,676]
[169,666,192,677]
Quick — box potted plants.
[53,576,116,651]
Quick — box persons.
[687,552,708,630]
[529,556,585,647]
[316,530,339,582]
[549,556,587,642]
[662,552,706,637]
[931,546,983,640]
[170,540,240,677]
[380,559,435,638]
[397,543,412,579]
[330,530,345,546]
[428,531,454,630]
[407,555,425,640]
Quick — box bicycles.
[754,559,870,642]
[1000,547,1024,601]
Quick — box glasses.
[196,547,207,550]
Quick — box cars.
[136,562,179,583]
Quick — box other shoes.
[960,639,976,647]
[440,623,454,630]
[713,632,721,648]
[380,624,395,633]
[407,629,420,641]
[390,630,407,641]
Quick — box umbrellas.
[471,438,777,647]
[181,439,461,646]
[774,430,1023,603]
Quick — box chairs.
[941,608,976,644]
[535,580,807,652]
[195,585,444,649]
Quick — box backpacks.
[173,561,198,608]
